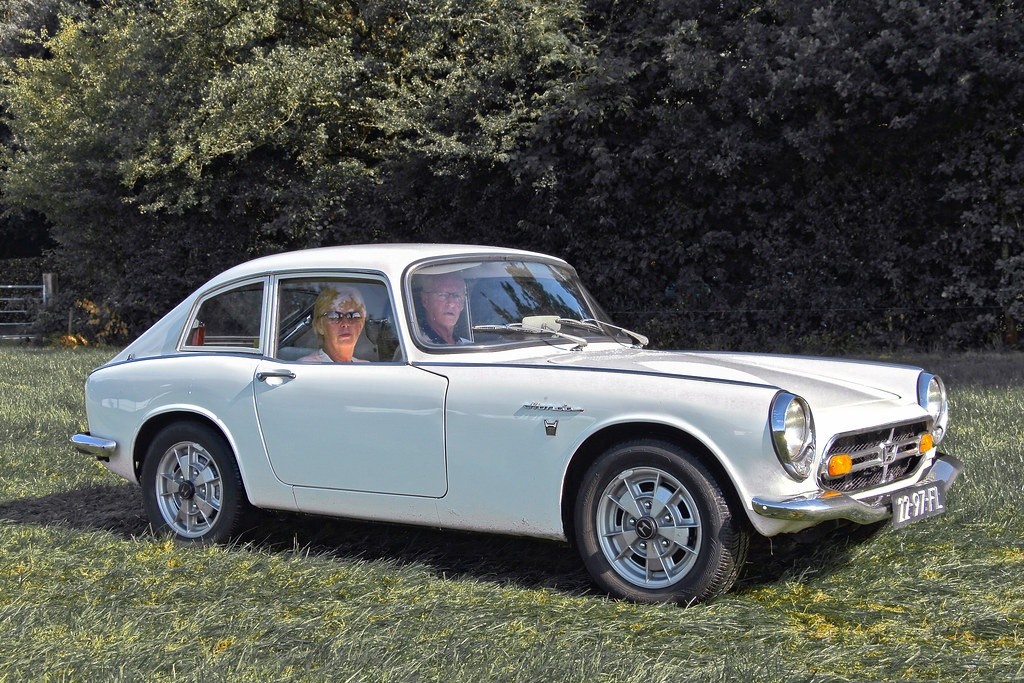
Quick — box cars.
[66,238,966,604]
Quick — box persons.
[294,285,369,362]
[391,270,475,362]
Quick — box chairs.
[296,313,379,364]
[368,298,400,362]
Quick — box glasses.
[317,310,362,324]
[422,292,461,303]
